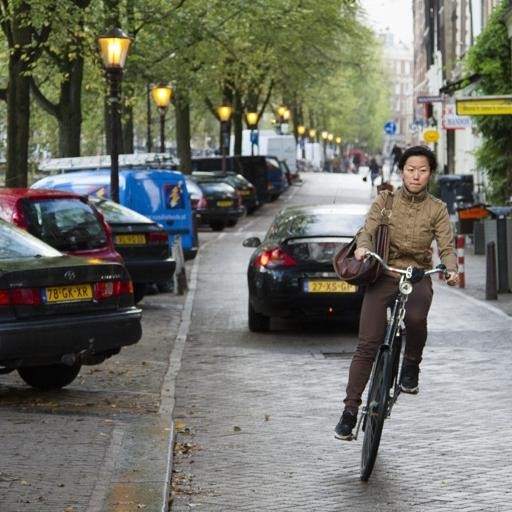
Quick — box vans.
[35,170,199,268]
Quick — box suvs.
[198,166,262,217]
[199,150,285,205]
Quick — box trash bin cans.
[483,207,510,294]
[437,174,474,234]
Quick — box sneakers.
[400,363,419,393]
[333,409,357,440]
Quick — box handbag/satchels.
[332,223,391,287]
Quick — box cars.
[0,183,135,310]
[0,214,145,410]
[241,205,447,333]
[179,172,247,238]
[77,188,176,317]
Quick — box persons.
[334,147,461,440]
[362,157,385,187]
[388,143,404,175]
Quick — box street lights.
[148,76,174,155]
[244,105,261,152]
[214,97,236,170]
[277,108,342,160]
[93,21,136,203]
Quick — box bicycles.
[344,242,461,478]
